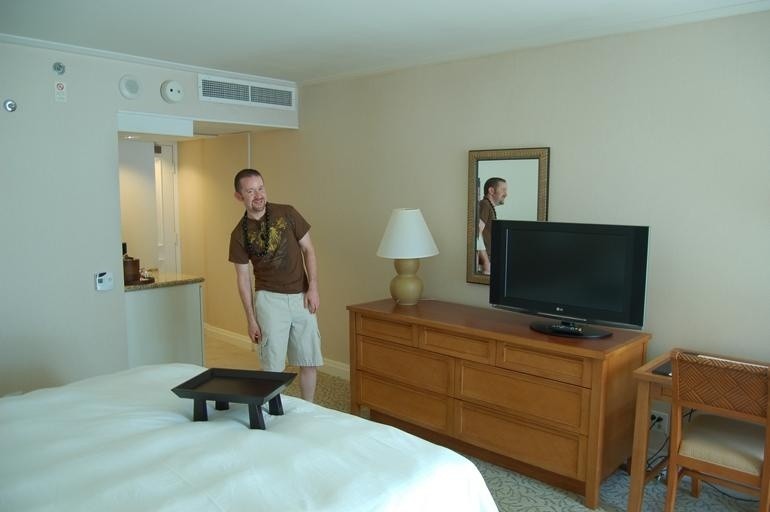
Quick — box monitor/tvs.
[489,218,649,340]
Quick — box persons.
[476,177,508,276]
[225,168,324,403]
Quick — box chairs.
[663,347,770,512]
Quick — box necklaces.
[484,197,497,219]
[242,201,271,257]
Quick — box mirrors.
[466,146,550,285]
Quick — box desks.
[627,349,770,512]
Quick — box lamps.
[375,207,439,306]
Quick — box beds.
[0,361,495,511]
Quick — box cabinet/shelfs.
[344,299,652,510]
